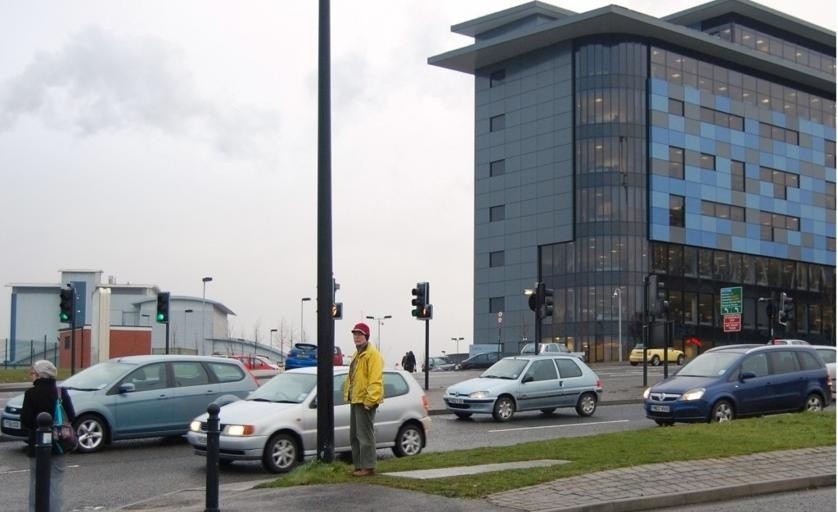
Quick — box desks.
[270,328,278,353]
[300,295,313,341]
[365,314,394,353]
[141,313,152,329]
[202,275,212,354]
[612,287,624,361]
[182,308,194,345]
[450,337,464,363]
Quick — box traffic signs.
[720,287,743,315]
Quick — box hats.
[628,339,835,427]
[421,341,604,423]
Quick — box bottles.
[351,323,369,335]
[32,359,57,379]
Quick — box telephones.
[720,287,743,315]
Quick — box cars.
[628,339,835,427]
[421,341,604,423]
[284,343,318,371]
[333,346,345,366]
[187,364,431,473]
[0,354,263,454]
[221,354,283,387]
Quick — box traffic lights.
[155,292,168,323]
[660,301,672,314]
[779,296,793,325]
[411,283,426,317]
[655,269,670,297]
[331,304,342,319]
[540,285,555,317]
[420,305,433,318]
[60,289,75,324]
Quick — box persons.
[343,323,384,477]
[404,351,415,371]
[19,359,75,511]
[401,352,408,370]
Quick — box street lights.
[612,287,624,361]
[270,328,278,353]
[300,295,313,341]
[182,308,194,345]
[51,388,79,454]
[141,313,152,329]
[202,275,212,354]
[365,314,394,353]
[450,337,464,363]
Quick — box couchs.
[540,285,555,317]
[779,296,793,325]
[660,301,672,314]
[411,283,426,317]
[420,305,433,318]
[155,292,168,323]
[655,269,670,297]
[60,289,75,324]
[331,304,342,319]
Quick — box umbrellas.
[352,468,375,476]
